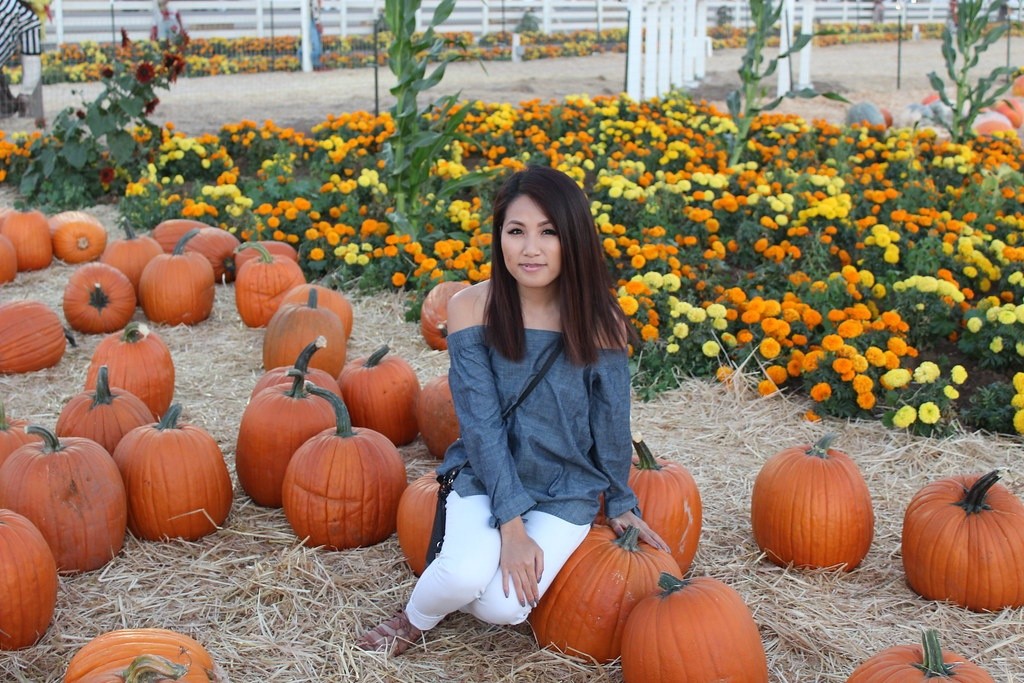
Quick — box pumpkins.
[526,432,1024,683]
[844,71,1024,143]
[0,201,464,683]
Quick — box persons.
[359,165,671,658]
[0,0,53,101]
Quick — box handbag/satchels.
[425,467,458,564]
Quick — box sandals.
[357,611,424,662]
